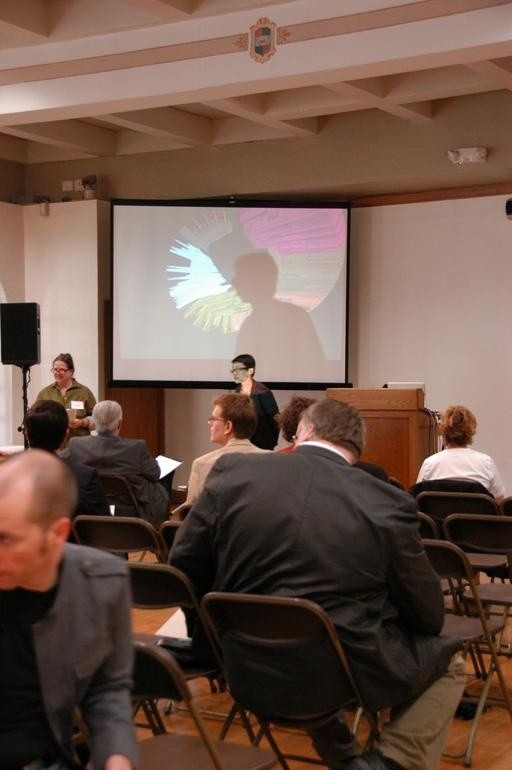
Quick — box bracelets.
[80,418,84,427]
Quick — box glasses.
[209,416,224,422]
[50,368,69,375]
[229,366,246,373]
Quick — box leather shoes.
[349,751,395,769]
[313,733,356,762]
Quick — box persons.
[413,404,505,587]
[230,354,281,451]
[168,398,467,769]
[1,400,69,463]
[0,450,139,770]
[232,252,329,383]
[69,399,175,530]
[185,394,273,507]
[273,395,318,454]
[36,353,95,454]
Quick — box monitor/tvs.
[386,382,427,390]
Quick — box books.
[152,605,193,650]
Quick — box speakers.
[0,302,41,366]
[505,197,512,218]
